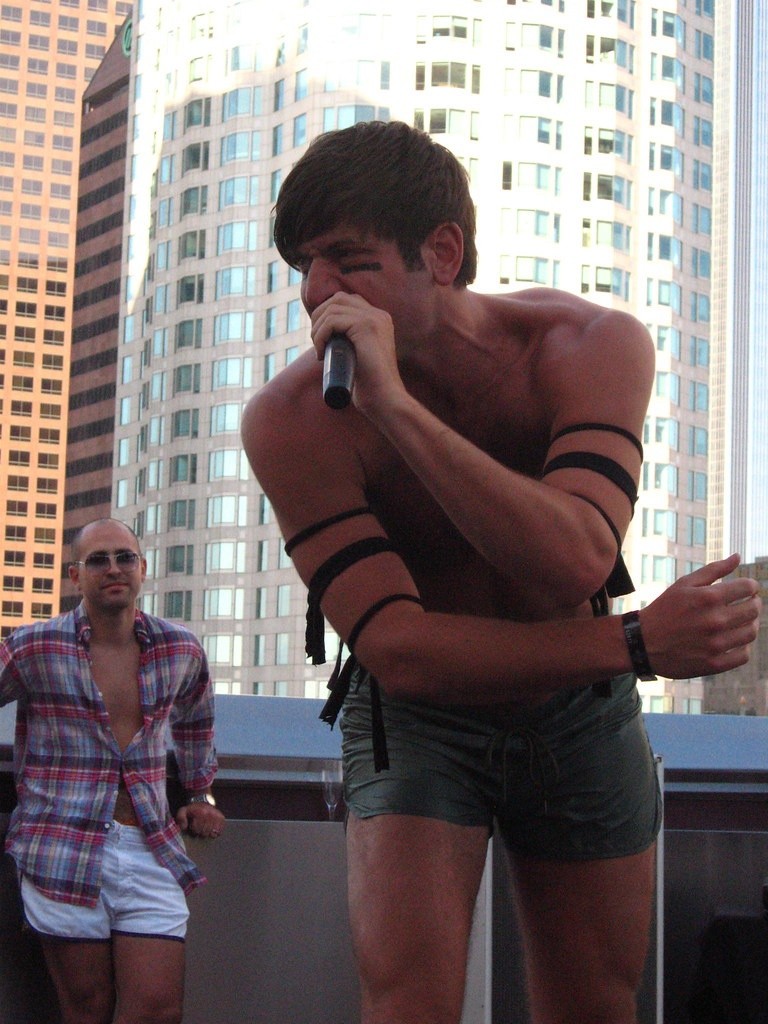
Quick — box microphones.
[322,332,357,410]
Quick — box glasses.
[74,551,140,572]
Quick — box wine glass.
[321,760,343,823]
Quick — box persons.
[242,121,761,1024]
[1,519,227,1024]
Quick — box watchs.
[187,794,216,806]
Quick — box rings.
[212,829,219,833]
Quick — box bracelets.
[622,611,657,682]
